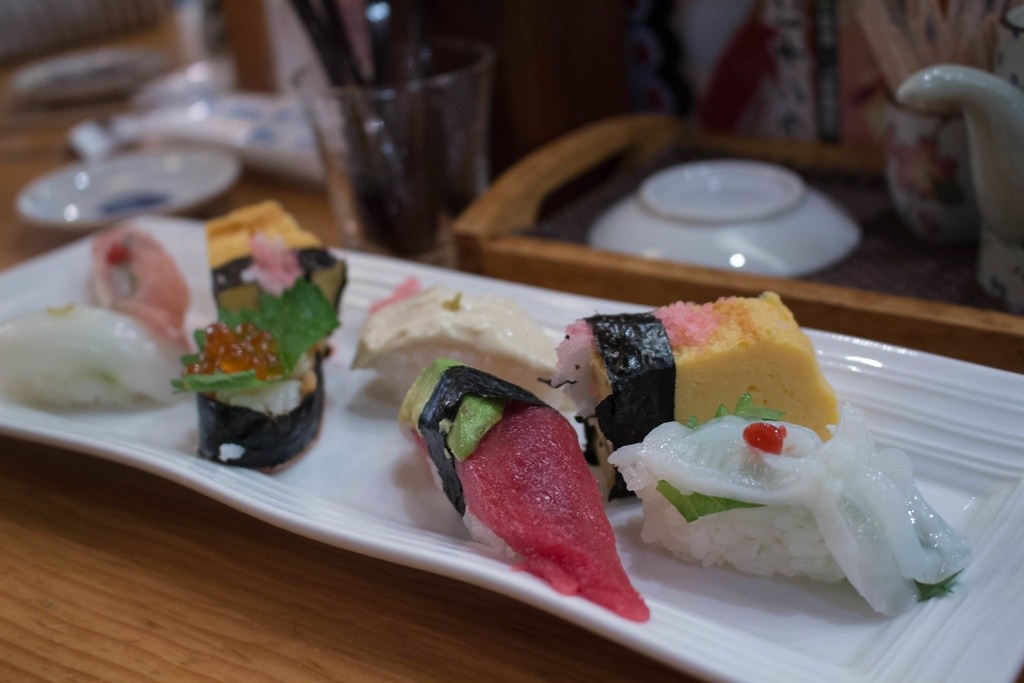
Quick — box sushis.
[0,201,974,626]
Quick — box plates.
[68,60,347,184]
[15,144,242,232]
[14,44,170,109]
[588,162,862,279]
[0,209,1024,683]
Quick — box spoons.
[288,0,481,254]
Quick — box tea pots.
[897,3,1024,314]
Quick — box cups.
[296,39,495,258]
[881,84,984,241]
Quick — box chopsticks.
[850,0,997,87]
